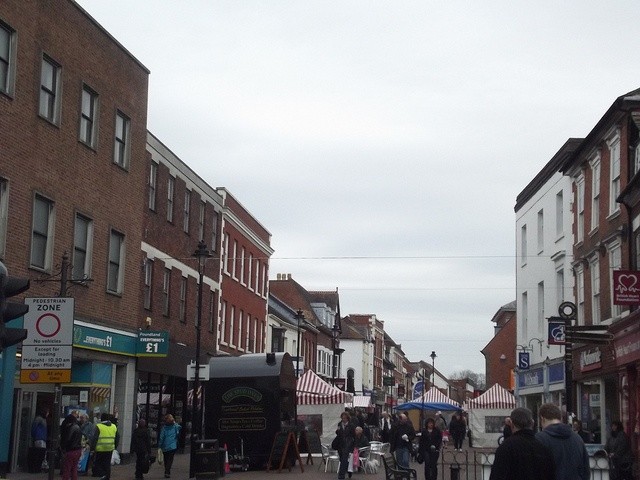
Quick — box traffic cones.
[223,443,231,473]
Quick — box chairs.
[358,441,391,473]
[321,443,340,473]
[381,453,417,480]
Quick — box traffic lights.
[0,276,30,349]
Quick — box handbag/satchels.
[332,422,342,449]
[158,448,164,463]
[347,448,360,473]
[142,456,156,473]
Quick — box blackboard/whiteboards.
[293,429,327,465]
[267,431,304,473]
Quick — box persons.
[63,409,80,424]
[449,414,460,453]
[108,414,119,427]
[178,417,187,453]
[535,403,590,480]
[419,418,442,480]
[90,413,120,480]
[503,417,512,435]
[389,413,416,480]
[458,414,466,452]
[131,419,152,480]
[403,411,413,426]
[354,426,370,472]
[604,420,634,480]
[434,411,449,449]
[59,419,83,480]
[28,407,50,473]
[92,413,101,421]
[157,413,182,478]
[489,406,556,480]
[380,411,391,443]
[572,420,589,443]
[333,412,354,480]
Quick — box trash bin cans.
[195,438,219,480]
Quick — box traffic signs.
[19,296,74,383]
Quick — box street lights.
[331,319,339,388]
[293,307,305,377]
[188,239,212,478]
[429,350,437,386]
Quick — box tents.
[296,370,354,457]
[468,383,517,448]
